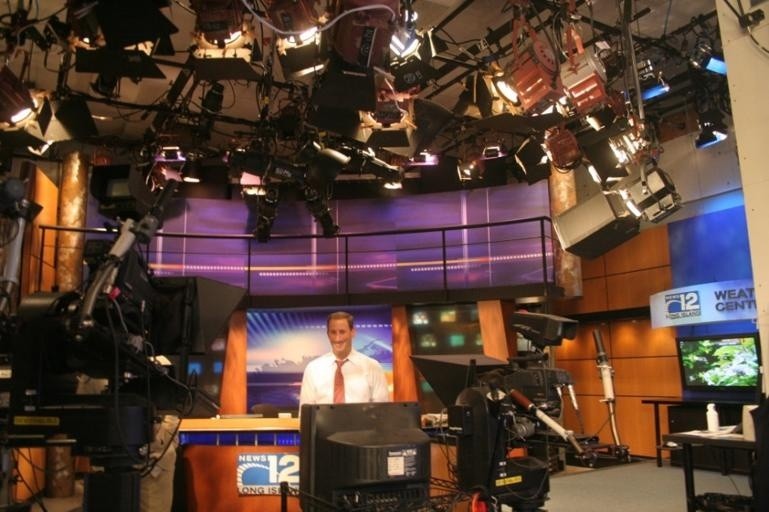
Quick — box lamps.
[0,0,729,242]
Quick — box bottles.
[706,403,718,432]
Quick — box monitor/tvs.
[298,401,432,511]
[676,332,762,391]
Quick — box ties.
[333,359,349,404]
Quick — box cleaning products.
[705,403,720,434]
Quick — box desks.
[641,396,759,467]
[662,431,769,512]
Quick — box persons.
[298,309,391,418]
